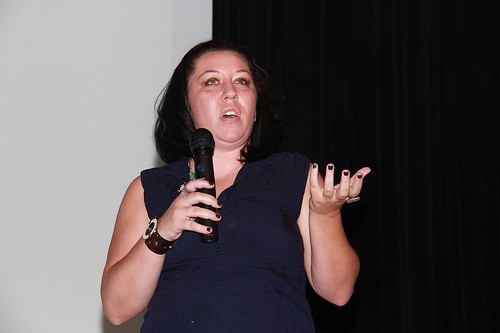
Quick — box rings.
[346,192,361,204]
[177,183,195,194]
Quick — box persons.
[100,39,371,333]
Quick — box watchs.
[143,216,181,255]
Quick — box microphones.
[189,128,219,243]
